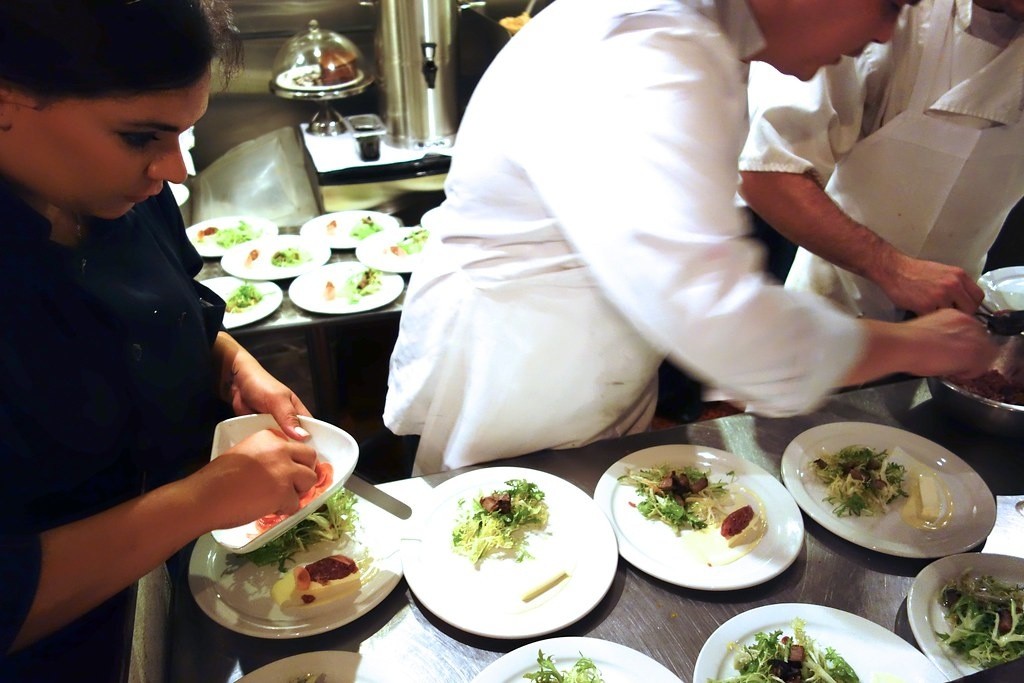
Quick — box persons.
[382,0,997,480]
[0,0,316,683]
[699,0,1024,417]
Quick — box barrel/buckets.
[374,1,459,148]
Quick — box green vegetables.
[241,482,360,576]
[615,464,736,536]
[454,477,549,565]
[215,216,428,312]
[808,444,911,518]
[704,616,860,683]
[933,566,1024,671]
[522,648,605,683]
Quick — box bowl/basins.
[926,374,1024,439]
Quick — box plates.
[401,466,619,639]
[189,485,404,638]
[594,443,804,592]
[781,421,997,559]
[977,265,1024,335]
[277,63,362,91]
[906,553,1024,681]
[233,649,391,683]
[468,638,685,683]
[164,178,443,330]
[693,603,949,683]
[209,414,365,554]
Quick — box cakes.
[317,48,359,86]
